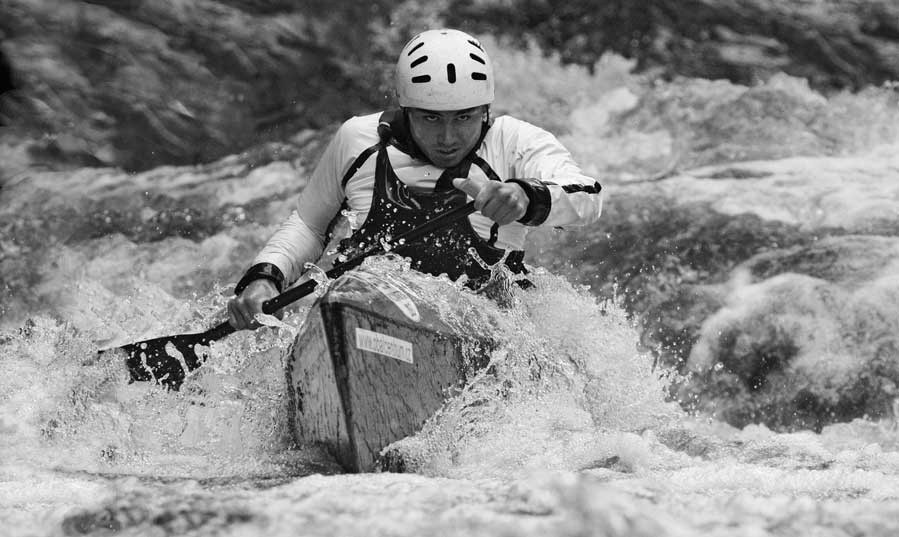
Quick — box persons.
[227,28,605,329]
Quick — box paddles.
[88,199,479,391]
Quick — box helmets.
[395,29,495,112]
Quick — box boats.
[286,271,514,476]
